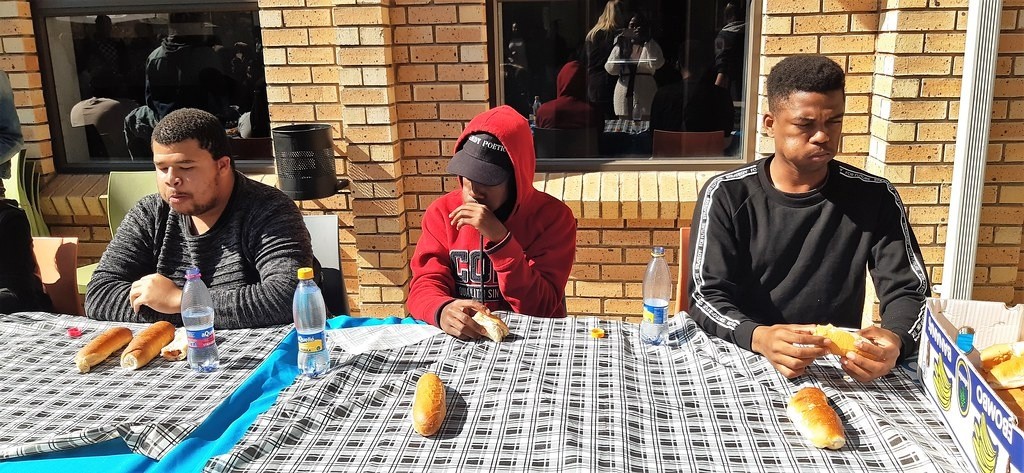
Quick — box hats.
[446,133,514,186]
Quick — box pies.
[160,338,188,361]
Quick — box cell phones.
[623,28,635,37]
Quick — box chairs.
[0,148,160,320]
[303,214,350,319]
[532,127,599,159]
[673,227,691,316]
[652,130,725,158]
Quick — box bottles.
[533,96,541,124]
[180,266,221,373]
[932,285,941,297]
[293,268,331,378]
[639,247,672,344]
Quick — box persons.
[0,67,52,314]
[83,107,334,328]
[506,0,745,153]
[406,105,576,339]
[81,12,270,161]
[688,53,932,381]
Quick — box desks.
[0,309,973,473]
[602,120,651,141]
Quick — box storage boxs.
[917,298,1024,473]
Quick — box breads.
[471,311,510,343]
[787,387,847,450]
[75,326,133,373]
[980,341,1024,390]
[813,323,876,358]
[411,373,447,436]
[121,321,176,369]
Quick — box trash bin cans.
[271,124,338,200]
